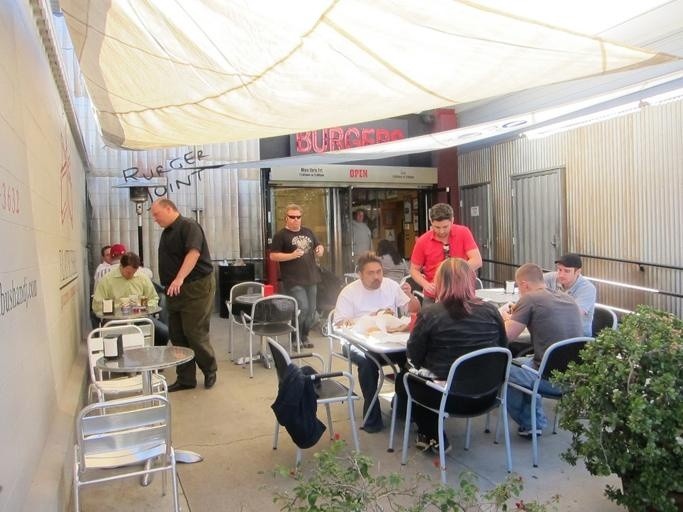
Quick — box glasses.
[286,215,301,220]
[443,243,450,259]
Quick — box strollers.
[313,266,348,338]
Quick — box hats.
[109,244,126,257]
[554,253,582,268]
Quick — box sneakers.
[517,425,543,438]
[292,335,314,349]
[414,430,453,456]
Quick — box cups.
[119,295,150,315]
[262,284,273,297]
[505,280,514,294]
[409,312,417,331]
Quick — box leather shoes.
[163,376,197,392]
[205,365,218,388]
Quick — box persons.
[333,253,422,434]
[544,253,597,337]
[505,261,585,436]
[93,244,154,293]
[91,251,170,377]
[344,209,372,268]
[377,237,411,282]
[150,198,218,391]
[269,203,325,351]
[394,256,509,452]
[95,246,112,272]
[410,202,483,306]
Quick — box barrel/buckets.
[218,262,255,318]
